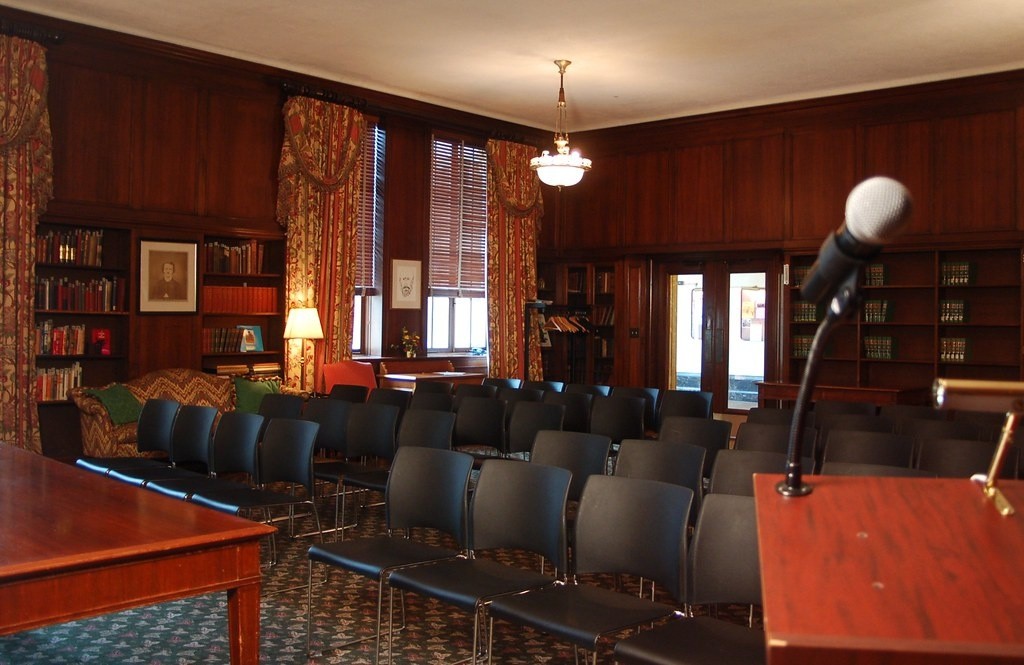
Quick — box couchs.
[65,367,310,460]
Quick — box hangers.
[540,311,601,336]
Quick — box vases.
[407,351,416,358]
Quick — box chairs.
[612,438,706,635]
[487,474,695,665]
[747,408,816,429]
[916,437,1021,480]
[613,494,766,665]
[818,413,895,456]
[822,428,917,470]
[75,377,715,596]
[706,448,816,497]
[733,423,819,460]
[306,446,482,665]
[884,404,956,422]
[812,399,883,438]
[323,360,377,401]
[990,425,1024,448]
[953,410,1024,426]
[658,415,733,494]
[386,459,579,665]
[891,414,983,441]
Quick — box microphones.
[801,176,914,304]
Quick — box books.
[203,325,264,353]
[204,285,277,313]
[35,360,83,401]
[205,239,264,274]
[33,318,110,356]
[33,273,126,313]
[791,262,977,286]
[567,271,615,294]
[792,297,970,322]
[203,360,281,380]
[594,337,617,357]
[592,302,615,324]
[792,335,970,361]
[34,228,104,267]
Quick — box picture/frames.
[389,257,424,312]
[135,236,200,316]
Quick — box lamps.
[283,307,324,390]
[528,60,592,192]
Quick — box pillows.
[234,377,281,414]
[82,383,143,425]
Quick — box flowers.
[390,325,422,355]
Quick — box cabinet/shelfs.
[36,215,137,409]
[782,242,1024,410]
[562,261,624,387]
[200,233,288,385]
[537,257,568,382]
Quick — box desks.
[754,381,930,409]
[376,372,488,396]
[0,441,278,665]
